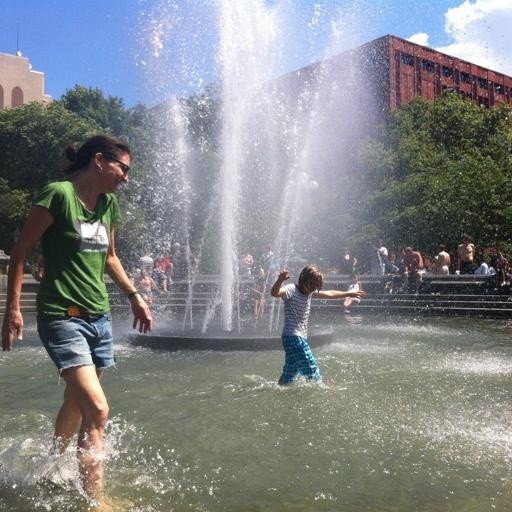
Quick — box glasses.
[102,154,131,174]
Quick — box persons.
[338,232,512,308]
[1,135,158,511]
[270,263,366,388]
[125,239,278,320]
